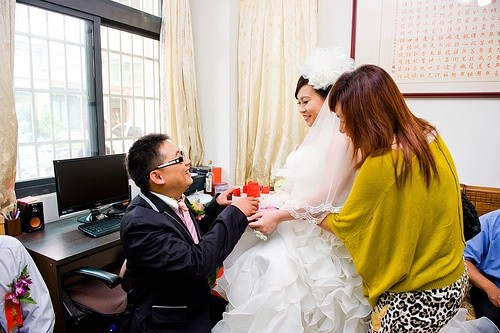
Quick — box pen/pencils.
[1,205,20,220]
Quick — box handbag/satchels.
[461,192,481,243]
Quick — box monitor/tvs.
[53,153,131,224]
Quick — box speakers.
[16,196,44,234]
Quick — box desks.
[16,214,120,320]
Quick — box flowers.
[4,263,38,333]
[186,194,205,221]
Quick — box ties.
[179,204,199,245]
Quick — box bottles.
[205,160,215,196]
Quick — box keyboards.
[77,214,124,237]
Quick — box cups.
[232,193,247,204]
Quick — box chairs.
[60,259,127,333]
[460,184,500,216]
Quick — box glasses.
[147,151,188,180]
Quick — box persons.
[318,64,469,333]
[119,134,260,333]
[0,235,55,333]
[213,49,374,333]
[463,208,500,330]
